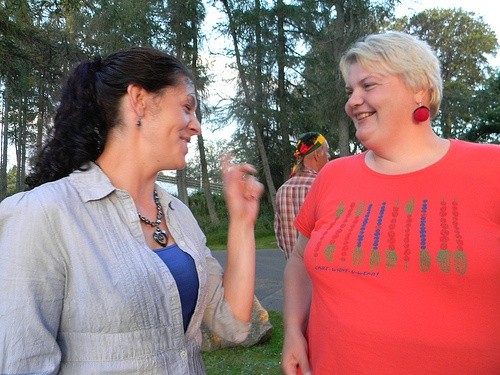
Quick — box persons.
[274,132,331,263]
[0,46,274,375]
[281,31,500,375]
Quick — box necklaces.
[133,189,172,248]
